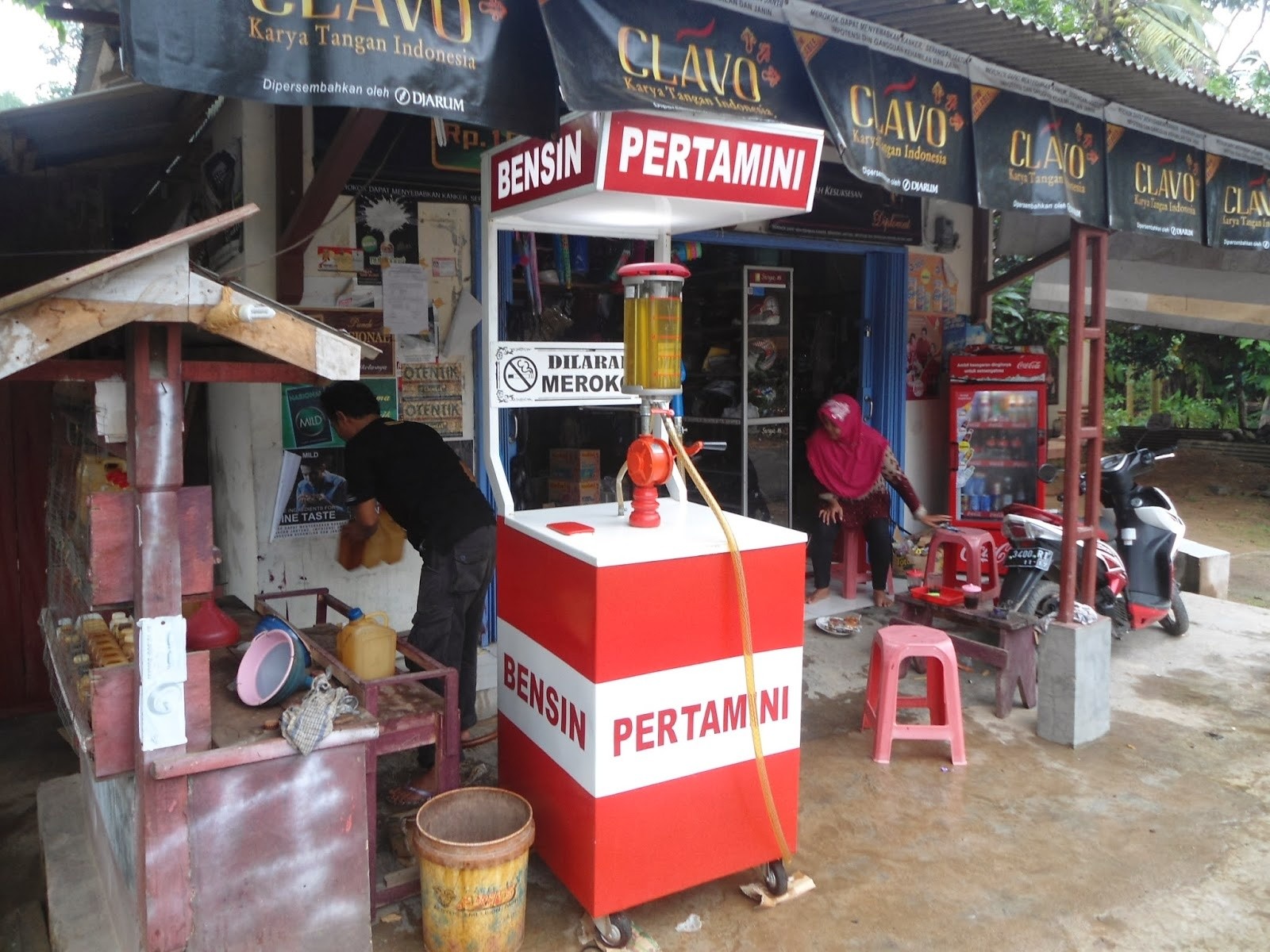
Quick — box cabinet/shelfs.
[682,264,792,527]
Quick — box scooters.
[987,409,1191,680]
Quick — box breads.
[82,619,134,666]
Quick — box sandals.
[387,782,431,808]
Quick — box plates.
[816,615,853,637]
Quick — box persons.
[806,393,953,608]
[318,381,499,805]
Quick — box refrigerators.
[948,354,1049,575]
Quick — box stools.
[923,525,1002,607]
[861,622,969,766]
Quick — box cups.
[926,571,944,597]
[906,568,924,592]
[961,584,982,609]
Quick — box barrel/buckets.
[411,786,537,952]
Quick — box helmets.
[730,336,778,372]
[699,381,740,413]
[749,384,777,405]
[747,296,781,325]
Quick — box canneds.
[961,472,1013,511]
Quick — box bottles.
[978,394,1037,422]
[984,432,1036,460]
[335,606,398,681]
[1001,476,1026,508]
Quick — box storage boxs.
[547,447,601,507]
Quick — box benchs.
[1171,537,1231,601]
[889,583,1041,718]
